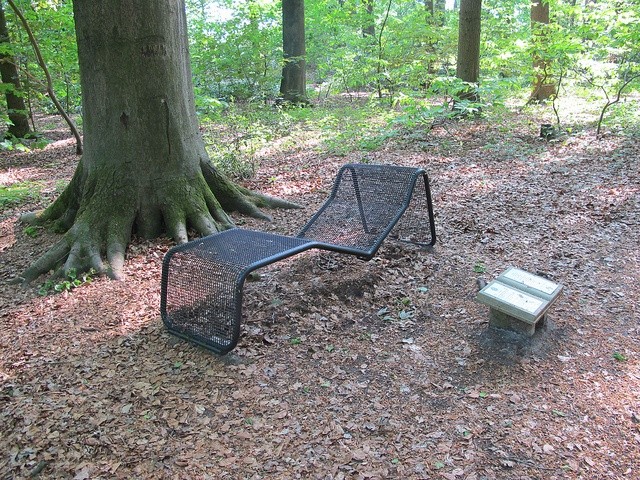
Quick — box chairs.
[158,164,438,356]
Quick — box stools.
[474,267,564,337]
